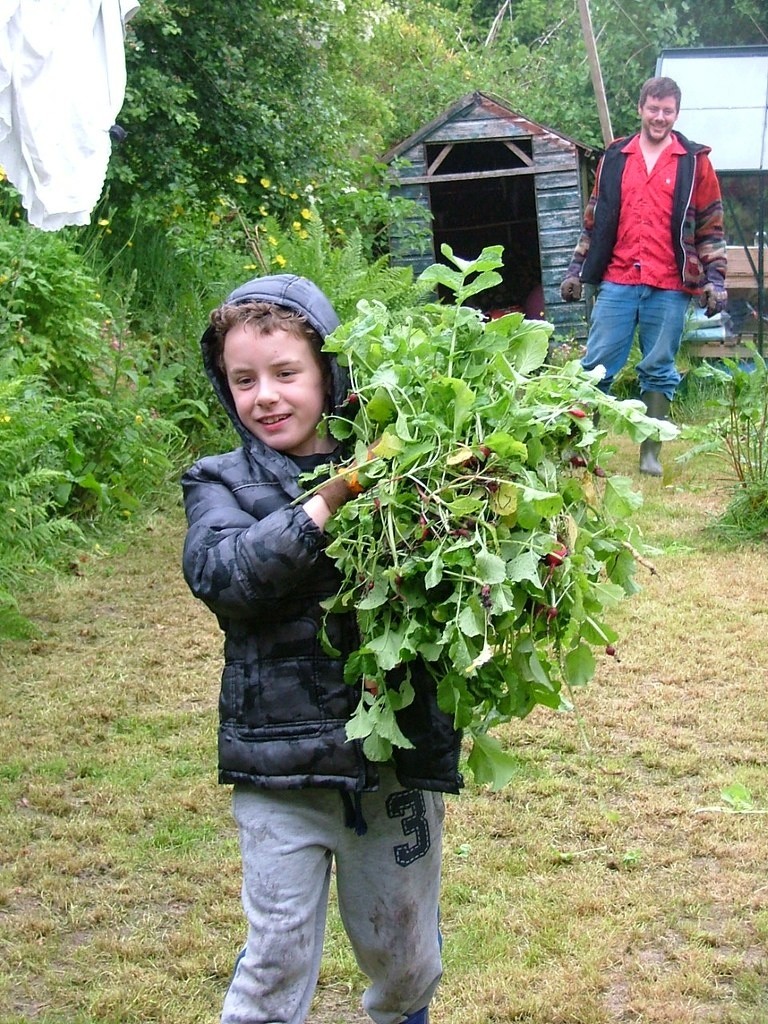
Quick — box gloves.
[561,277,582,302]
[698,282,727,316]
[312,437,383,514]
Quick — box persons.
[176,273,464,1023]
[559,77,727,478]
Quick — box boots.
[570,381,613,437]
[639,390,671,476]
